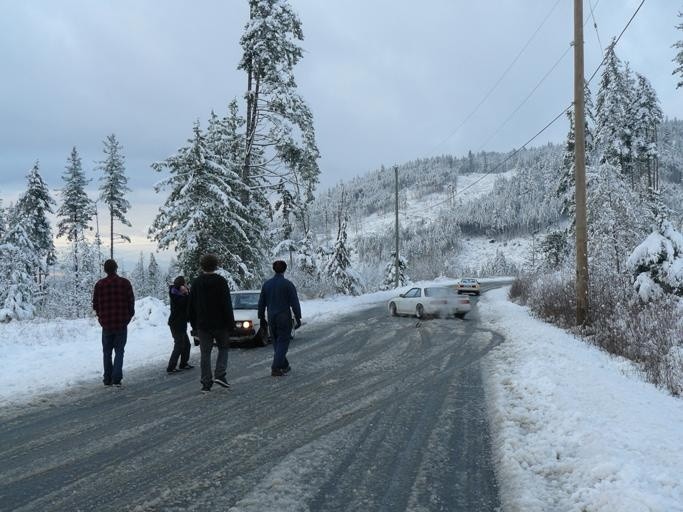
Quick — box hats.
[173,276,190,286]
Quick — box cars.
[387,278,480,319]
[191,290,296,348]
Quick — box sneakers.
[283,366,291,373]
[271,371,283,376]
[215,375,231,387]
[179,362,194,369]
[201,388,213,392]
[166,368,182,374]
[103,380,122,388]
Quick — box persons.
[166,274,193,373]
[258,261,302,376]
[188,255,236,394]
[93,259,135,388]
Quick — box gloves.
[294,319,301,329]
[260,319,267,327]
